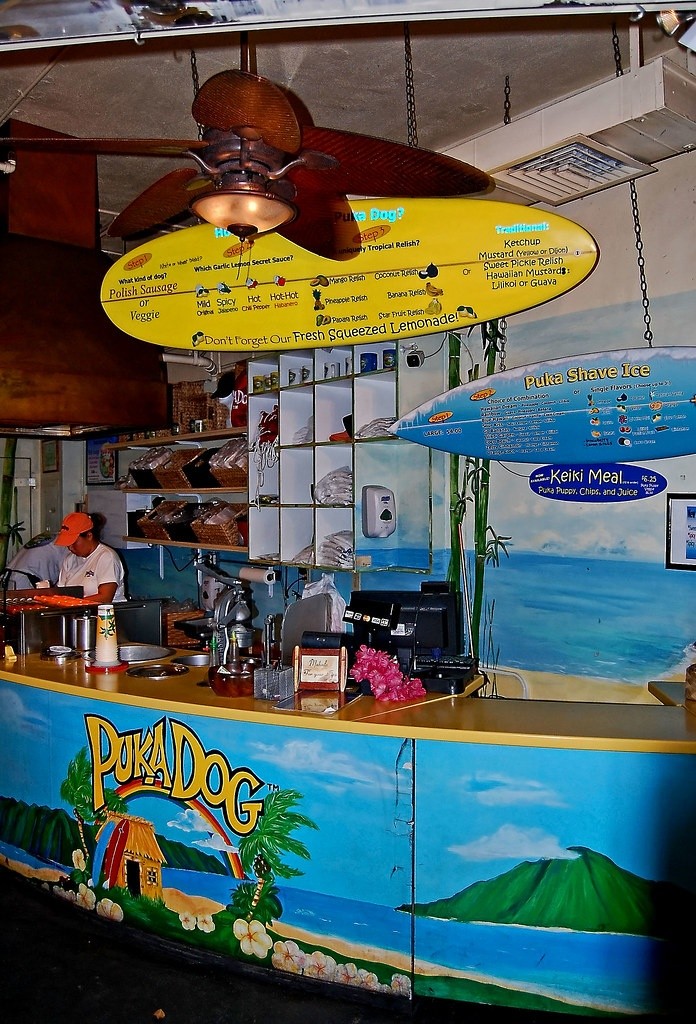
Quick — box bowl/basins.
[230,628,255,647]
[208,663,257,697]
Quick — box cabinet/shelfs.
[119,332,450,574]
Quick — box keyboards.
[413,655,481,671]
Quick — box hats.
[53,513,93,547]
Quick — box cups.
[253,371,279,393]
[302,365,313,382]
[360,353,377,372]
[288,368,301,385]
[71,610,95,650]
[96,605,119,663]
[324,362,340,378]
[346,357,353,375]
[383,349,397,368]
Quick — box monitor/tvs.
[385,590,464,676]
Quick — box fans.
[1,31,492,256]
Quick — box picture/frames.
[41,439,60,472]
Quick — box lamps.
[190,182,296,241]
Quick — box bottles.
[229,631,239,663]
[210,635,219,667]
[171,423,180,435]
[189,420,203,433]
[145,431,155,439]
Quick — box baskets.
[162,610,205,646]
[126,375,251,546]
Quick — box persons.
[0,533,70,590]
[53,513,127,604]
[211,371,235,428]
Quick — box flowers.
[350,647,426,702]
